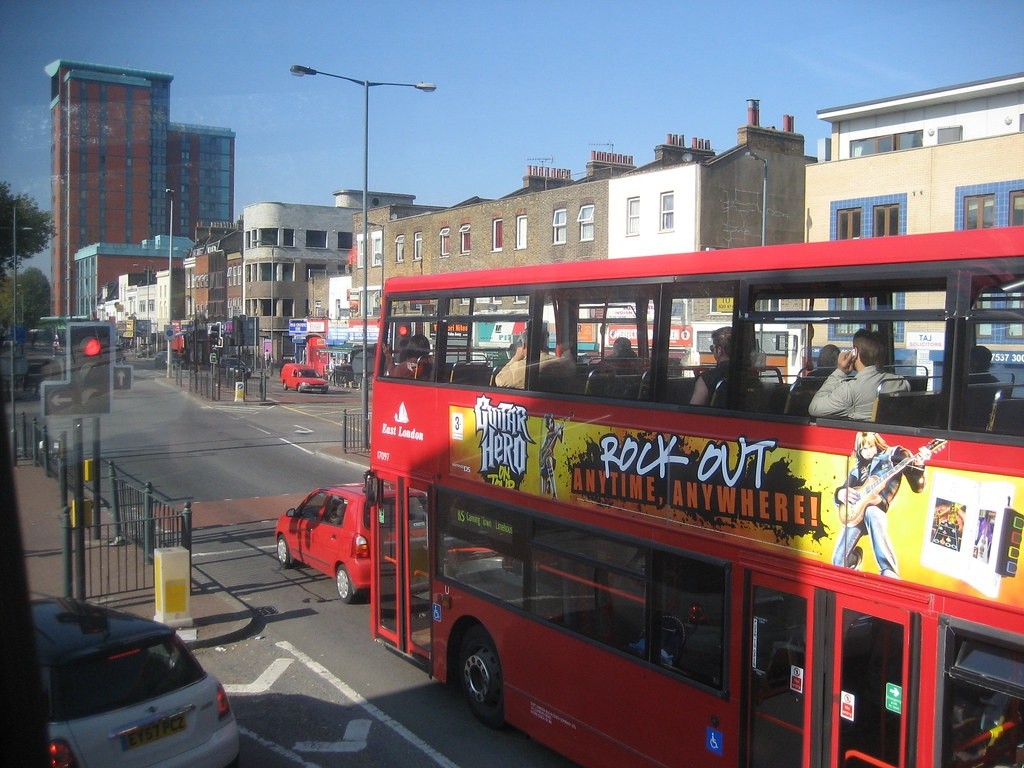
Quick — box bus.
[363,224,1024,768]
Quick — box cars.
[220,358,251,378]
[154,351,188,369]
[31,598,240,768]
[274,483,428,604]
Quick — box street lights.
[290,64,437,445]
[253,239,274,371]
[133,264,150,358]
[166,187,175,378]
[746,152,767,353]
[14,205,39,344]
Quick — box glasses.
[710,344,722,352]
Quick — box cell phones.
[846,349,856,357]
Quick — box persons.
[330,499,342,514]
[264,349,271,370]
[624,618,683,667]
[808,328,911,422]
[494,325,556,390]
[974,514,993,563]
[329,359,347,387]
[968,345,1000,384]
[604,337,639,358]
[382,334,433,380]
[689,327,765,408]
[52,340,59,357]
[124,339,128,347]
[121,356,126,366]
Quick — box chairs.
[412,352,1024,435]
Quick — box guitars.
[537,409,575,469]
[838,436,948,527]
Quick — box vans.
[281,363,329,394]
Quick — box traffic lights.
[209,322,223,348]
[392,322,416,357]
[235,321,244,346]
[41,322,133,418]
